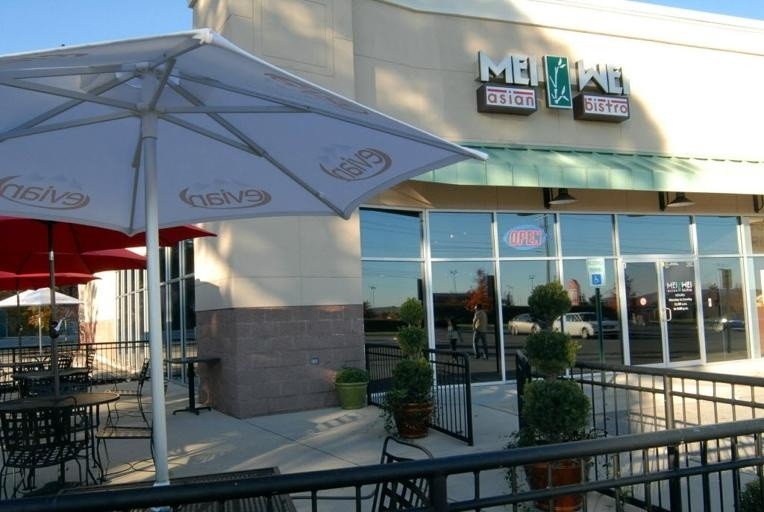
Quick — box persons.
[471,303,491,360]
[445,318,465,354]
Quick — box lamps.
[667,192,696,208]
[548,188,578,204]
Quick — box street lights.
[529,272,534,290]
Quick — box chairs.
[290,435,434,512]
[0,348,157,500]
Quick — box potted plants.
[334,367,370,410]
[500,282,632,512]
[377,297,444,445]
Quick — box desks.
[52,465,297,512]
[164,356,220,416]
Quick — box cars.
[508,311,620,339]
[712,318,744,332]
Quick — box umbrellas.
[0,212,217,401]
[1,269,103,375]
[1,26,490,484]
[0,287,85,355]
[0,247,148,396]
